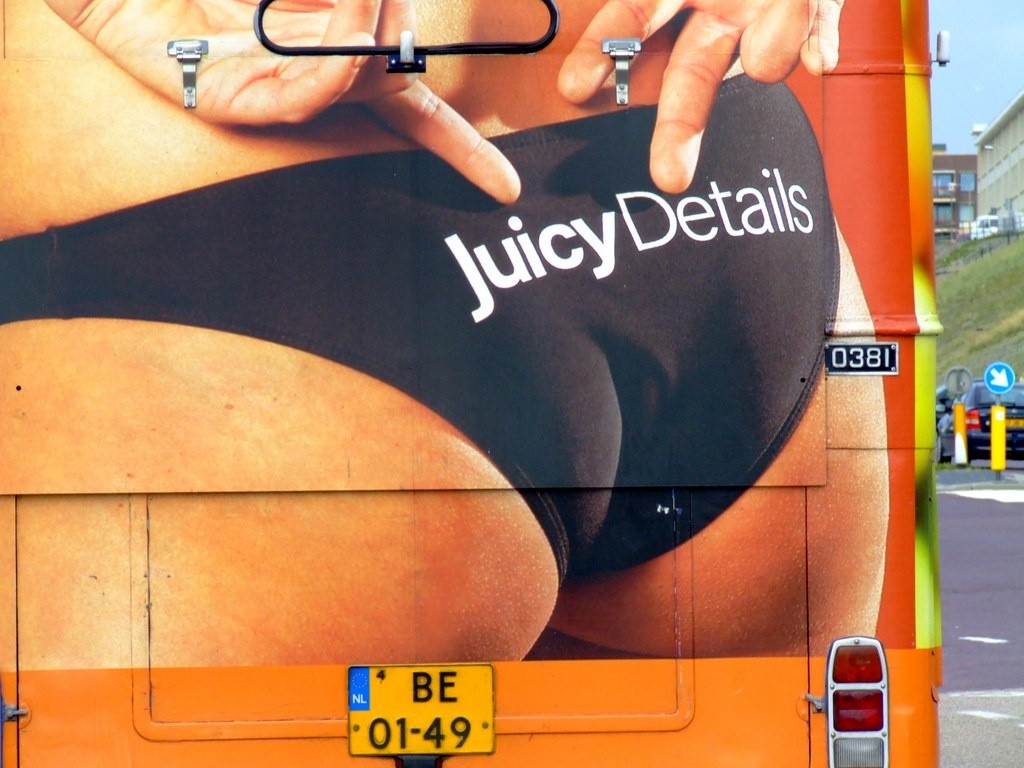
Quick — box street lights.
[983,145,1022,212]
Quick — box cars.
[935,377,1024,464]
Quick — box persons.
[1,1,891,681]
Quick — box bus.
[0,0,945,768]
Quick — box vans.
[970,214,999,241]
